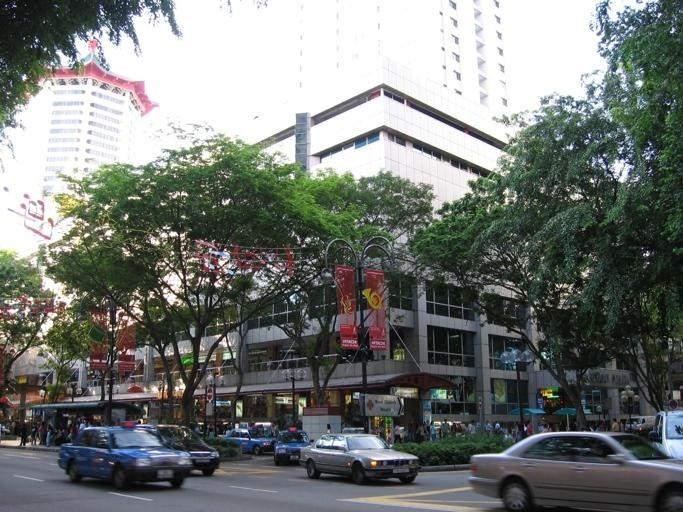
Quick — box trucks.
[624,416,655,431]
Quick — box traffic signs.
[365,395,403,416]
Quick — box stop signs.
[478,398,482,408]
[206,387,213,401]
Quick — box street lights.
[38,386,48,403]
[595,405,601,425]
[157,379,166,423]
[619,384,639,432]
[106,385,119,395]
[65,385,82,403]
[499,350,534,440]
[281,368,306,418]
[205,372,225,438]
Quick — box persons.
[394,420,647,444]
[2,419,302,448]
[325,424,330,433]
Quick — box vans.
[197,423,215,437]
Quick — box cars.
[156,424,219,477]
[467,432,683,512]
[301,434,421,485]
[57,420,193,490]
[274,428,314,466]
[222,429,274,455]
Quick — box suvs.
[647,411,683,458]
